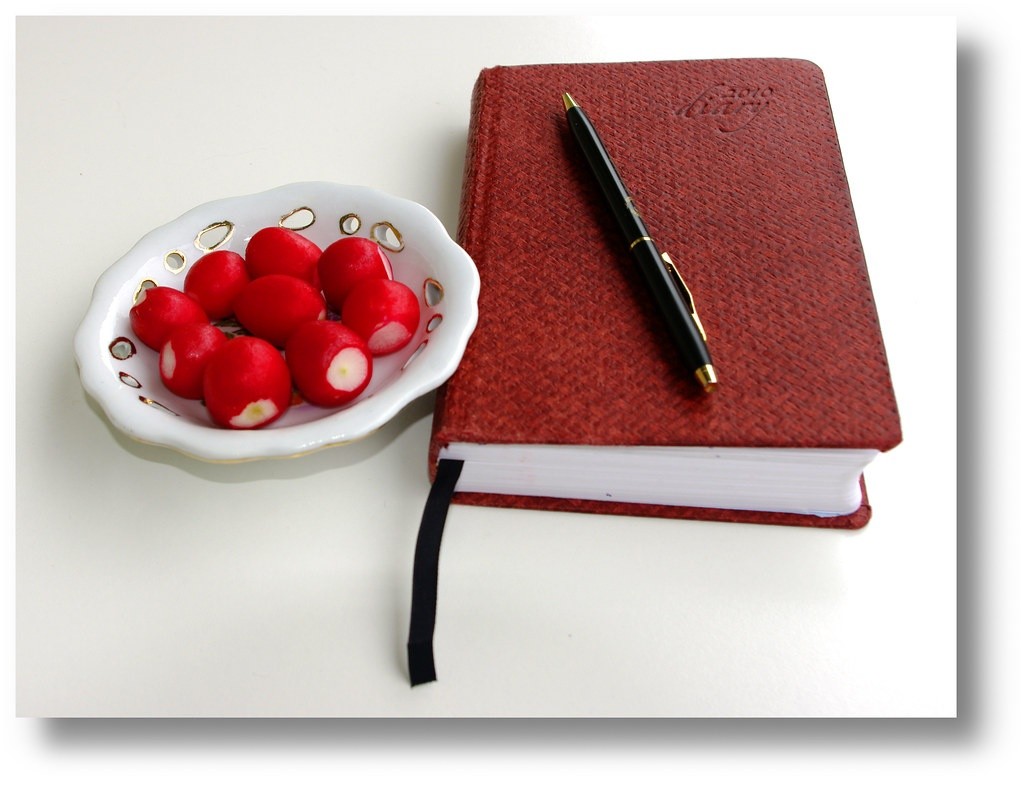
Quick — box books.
[425,56,905,528]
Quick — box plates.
[72,180,481,464]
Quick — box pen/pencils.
[561,92,718,394]
